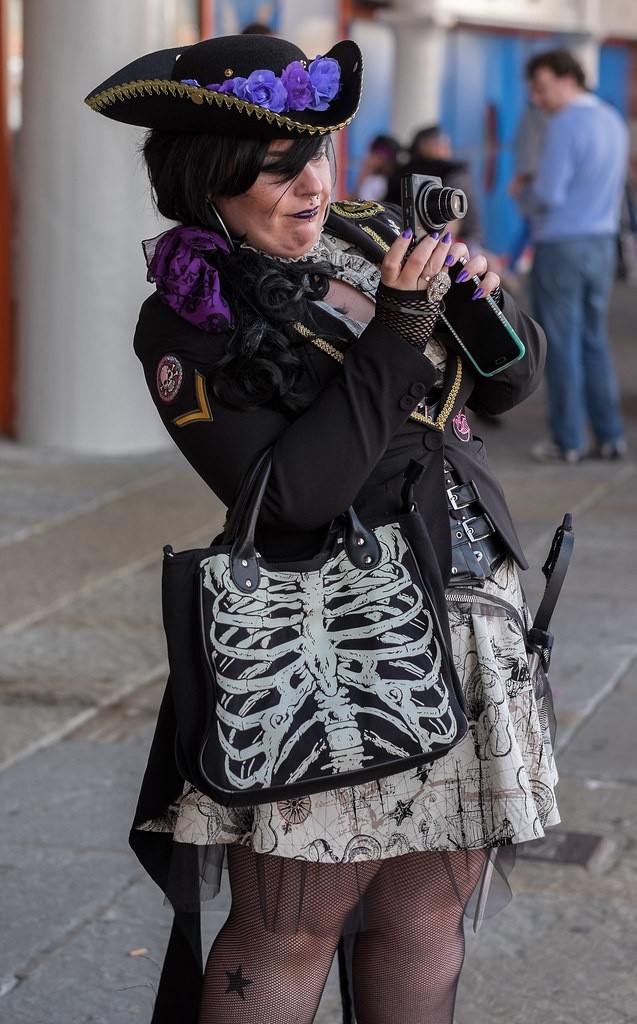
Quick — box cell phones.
[437,256,526,377]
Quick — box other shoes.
[588,440,629,460]
[531,438,588,465]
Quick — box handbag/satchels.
[161,446,468,807]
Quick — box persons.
[508,47,631,463]
[83,35,562,1024]
[356,125,477,241]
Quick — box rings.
[420,272,451,303]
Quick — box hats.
[84,35,364,136]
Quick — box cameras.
[400,173,468,251]
